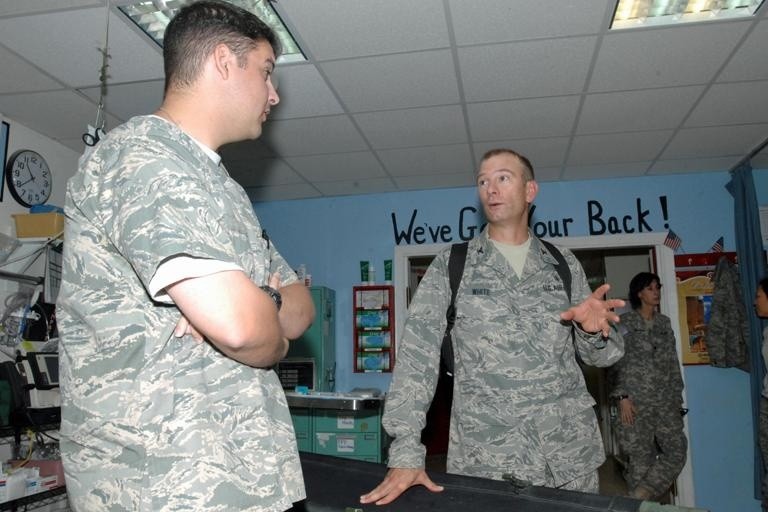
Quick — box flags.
[664,229,681,252]
[711,236,724,252]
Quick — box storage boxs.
[11,212,64,239]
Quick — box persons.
[359,150,626,506]
[604,272,688,503]
[55,2,316,512]
[752,276,768,512]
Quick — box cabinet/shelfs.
[279,286,336,393]
[353,285,395,373]
[288,406,312,454]
[312,406,392,464]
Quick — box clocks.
[6,149,53,208]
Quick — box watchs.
[615,395,628,401]
[258,284,283,313]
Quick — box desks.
[296,449,704,512]
